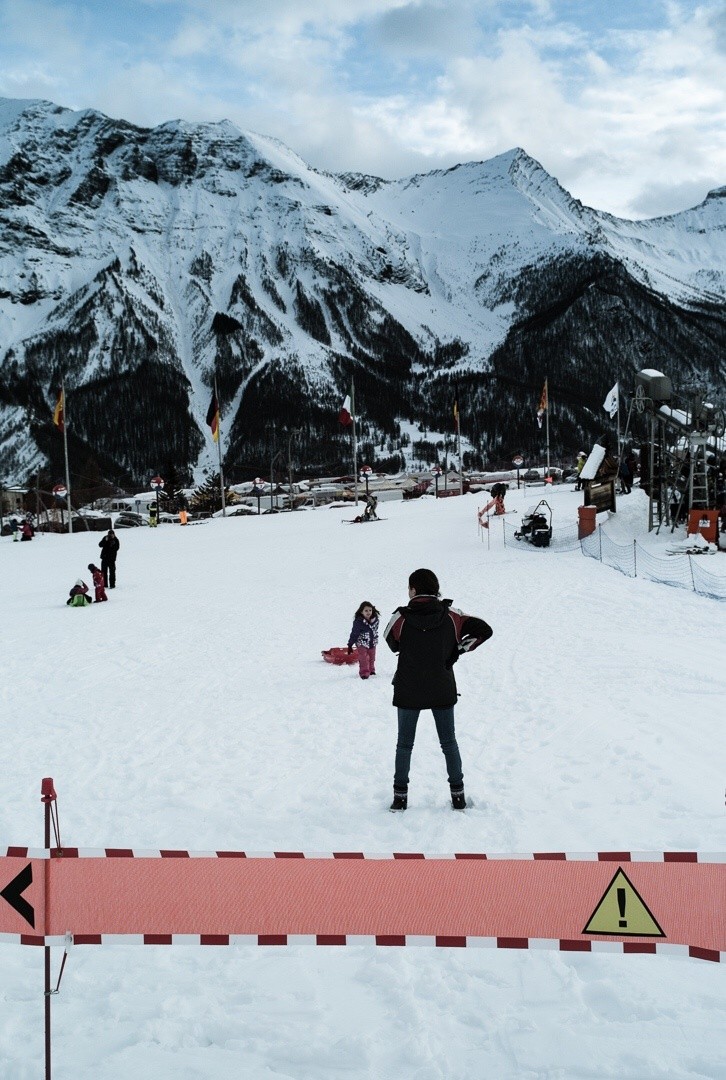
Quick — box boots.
[390,785,408,811]
[450,782,467,810]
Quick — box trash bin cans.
[578,506,596,539]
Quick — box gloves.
[347,647,353,655]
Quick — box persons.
[619,454,637,494]
[478,483,509,528]
[343,486,354,502]
[146,501,159,527]
[10,519,35,542]
[383,569,494,812]
[347,600,381,680]
[362,495,378,520]
[88,564,108,603]
[99,530,119,589]
[176,494,189,526]
[577,455,587,490]
[69,579,88,603]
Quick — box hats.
[75,578,83,587]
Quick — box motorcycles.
[513,501,553,548]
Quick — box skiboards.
[487,509,517,518]
[340,518,389,526]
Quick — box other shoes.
[362,676,368,680]
[370,672,376,675]
[94,598,107,602]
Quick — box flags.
[454,399,459,421]
[339,385,352,425]
[206,380,219,442]
[537,382,547,428]
[603,383,619,419]
[53,387,64,434]
